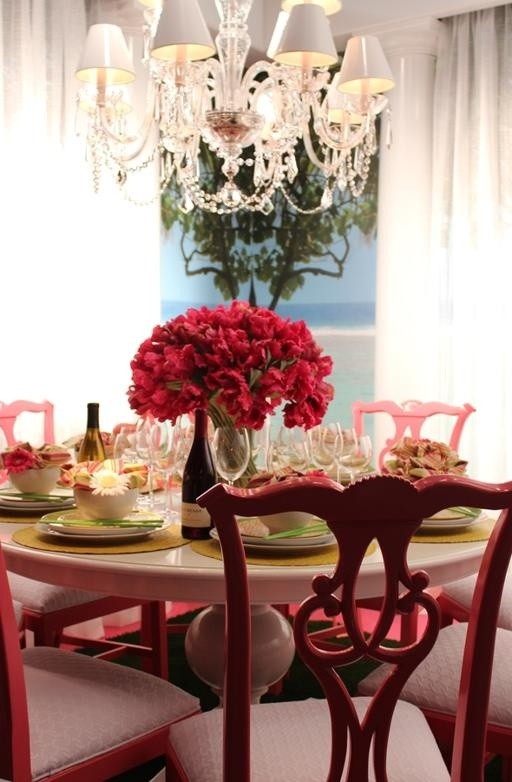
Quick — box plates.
[419,506,489,533]
[34,510,173,543]
[0,486,77,512]
[208,517,337,551]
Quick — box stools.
[353,619,511,782]
[436,570,510,628]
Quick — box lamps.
[74,1,393,225]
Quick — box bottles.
[181,406,219,540]
[78,403,106,461]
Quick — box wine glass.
[113,415,251,520]
[266,422,373,487]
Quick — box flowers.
[127,301,334,476]
[4,447,38,474]
[89,470,132,498]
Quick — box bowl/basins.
[74,487,139,521]
[257,511,314,534]
[9,467,61,497]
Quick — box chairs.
[0,533,200,782]
[161,472,510,781]
[1,399,168,683]
[346,400,475,612]
